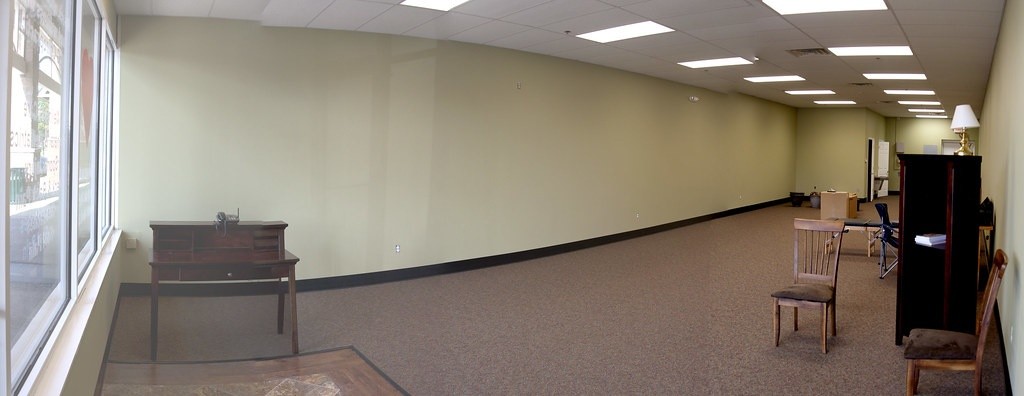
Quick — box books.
[915,233,946,246]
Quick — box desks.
[148,246,300,361]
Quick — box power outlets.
[126,238,137,249]
[814,186,816,189]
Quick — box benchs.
[824,217,899,258]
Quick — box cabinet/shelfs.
[895,154,983,346]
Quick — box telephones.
[216,207,240,223]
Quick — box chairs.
[874,203,898,279]
[771,218,845,353]
[904,249,1009,396]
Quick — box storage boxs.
[821,191,849,220]
[849,193,857,219]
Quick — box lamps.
[951,104,980,155]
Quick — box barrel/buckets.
[790,192,804,207]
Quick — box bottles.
[809,185,819,208]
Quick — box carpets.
[94,345,411,396]
